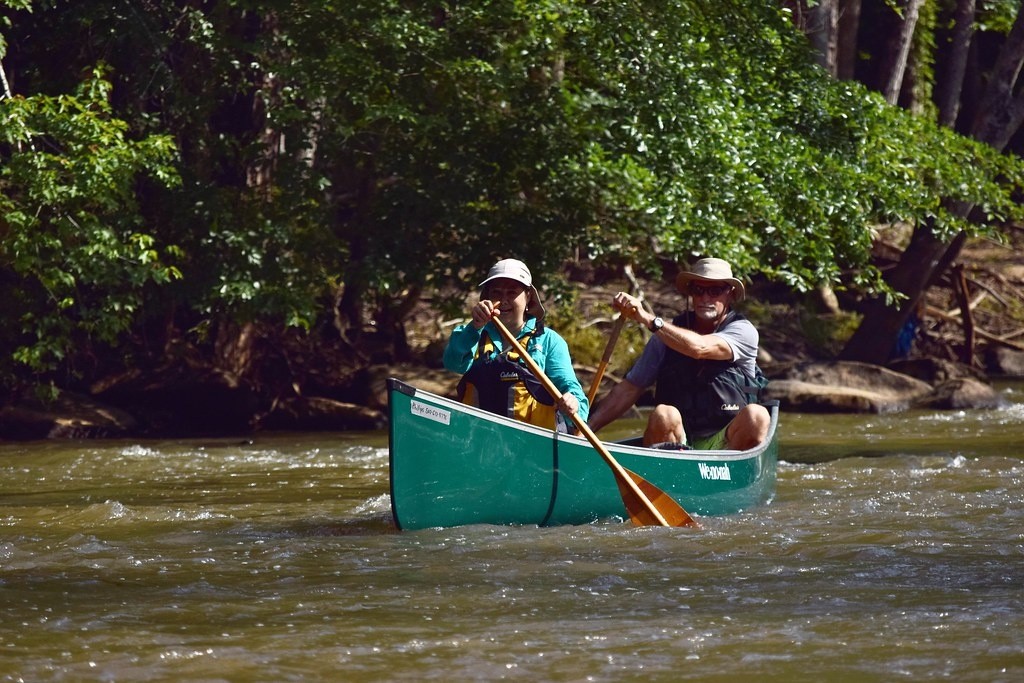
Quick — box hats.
[478,258,545,322]
[675,257,745,304]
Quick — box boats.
[384,374,781,535]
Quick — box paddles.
[574,299,637,436]
[476,299,698,527]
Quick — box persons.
[441,258,589,434]
[578,256,771,454]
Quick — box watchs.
[648,316,664,333]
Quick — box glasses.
[690,284,729,298]
